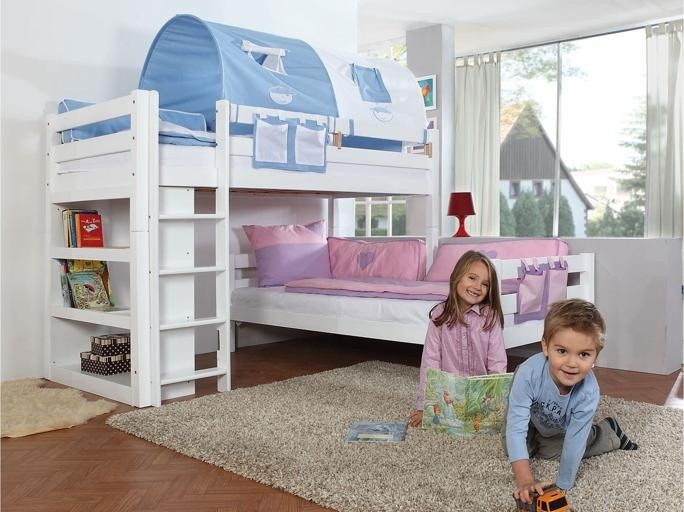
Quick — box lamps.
[447,191,477,238]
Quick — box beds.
[42,88,596,409]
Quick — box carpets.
[102,358,684,512]
[2,378,120,439]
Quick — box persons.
[499,297,639,504]
[408,248,508,429]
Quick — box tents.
[139,13,428,154]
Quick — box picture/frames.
[410,74,438,154]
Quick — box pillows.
[326,236,425,281]
[59,98,206,145]
[243,220,330,285]
[425,237,567,283]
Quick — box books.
[61,208,104,248]
[344,420,408,443]
[59,259,129,313]
[421,367,513,442]
